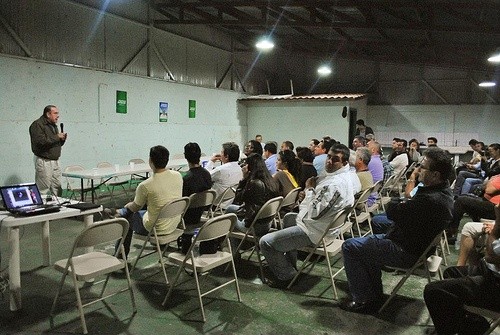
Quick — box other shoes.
[447,237,455,245]
[264,277,290,288]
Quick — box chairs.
[128,158,149,189]
[284,157,450,313]
[65,165,98,202]
[224,196,283,283]
[161,213,242,323]
[206,183,238,218]
[49,218,138,334]
[97,162,129,202]
[179,190,217,229]
[130,197,191,285]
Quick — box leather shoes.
[337,298,375,311]
[334,279,354,292]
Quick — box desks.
[62,156,212,204]
[0,194,104,311]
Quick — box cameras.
[481,145,489,156]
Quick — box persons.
[104,146,184,274]
[203,142,243,212]
[29,105,67,197]
[182,141,212,254]
[239,118,500,335]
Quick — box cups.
[130,162,135,169]
[114,165,119,172]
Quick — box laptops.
[0,184,61,216]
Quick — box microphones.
[60,123,63,133]
